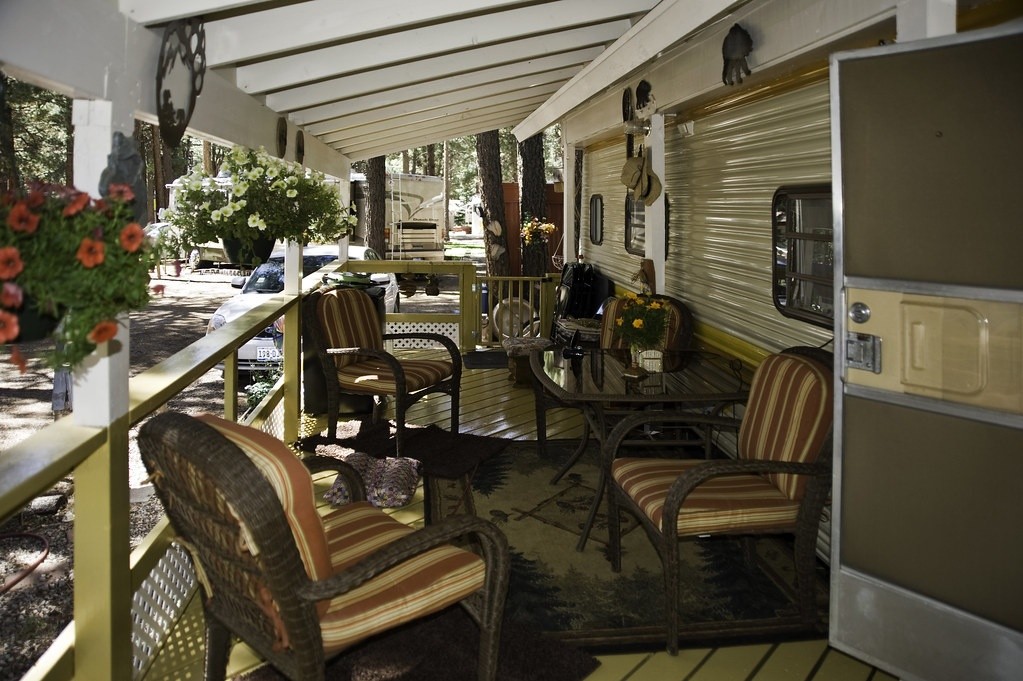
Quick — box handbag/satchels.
[556,262,595,319]
[322,271,380,290]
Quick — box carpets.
[426,440,829,655]
[240,596,604,681]
[366,423,512,480]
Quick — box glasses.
[562,345,584,359]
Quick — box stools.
[500,336,565,387]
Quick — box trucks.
[164,170,446,271]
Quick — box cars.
[141,222,186,262]
[202,245,401,384]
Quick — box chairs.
[138,412,511,681]
[602,345,834,656]
[536,294,692,459]
[308,282,462,458]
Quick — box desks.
[529,346,756,552]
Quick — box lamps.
[624,120,651,136]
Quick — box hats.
[620,146,649,202]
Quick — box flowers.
[0,145,161,373]
[614,287,673,352]
[520,214,557,256]
[157,144,359,255]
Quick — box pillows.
[323,452,421,505]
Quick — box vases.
[630,341,651,367]
[222,232,277,265]
[0,294,64,340]
